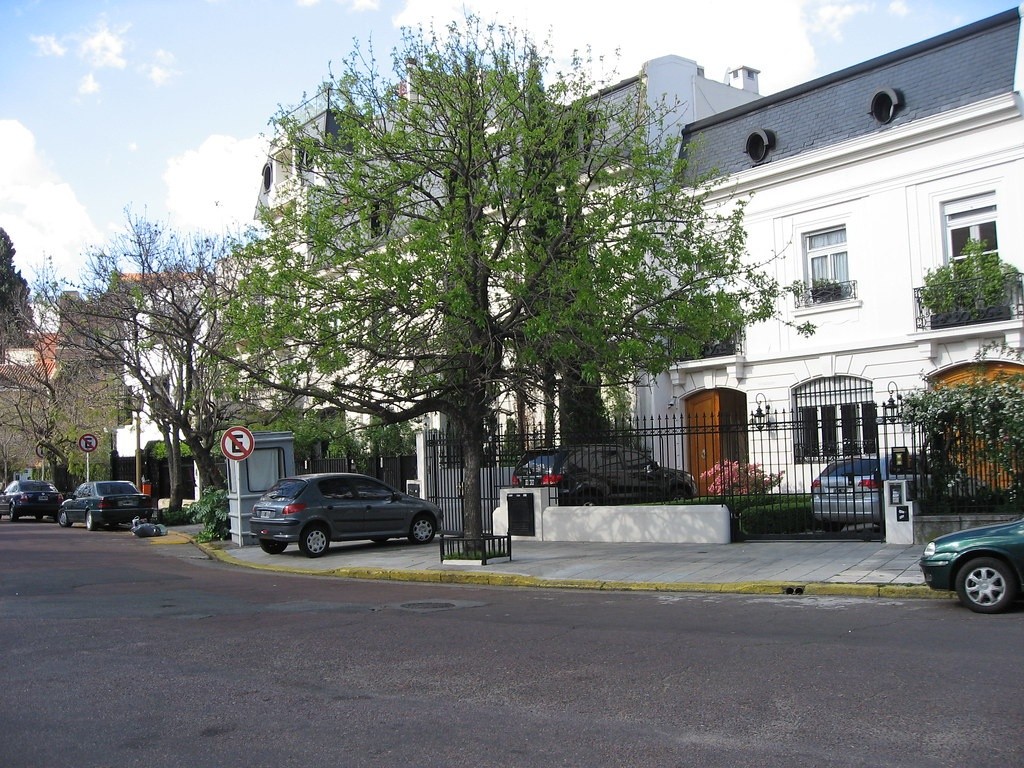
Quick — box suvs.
[810,453,985,533]
[512,442,697,506]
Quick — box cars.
[57,480,154,531]
[131,386,144,491]
[919,516,1024,614]
[0,480,63,523]
[249,472,442,558]
